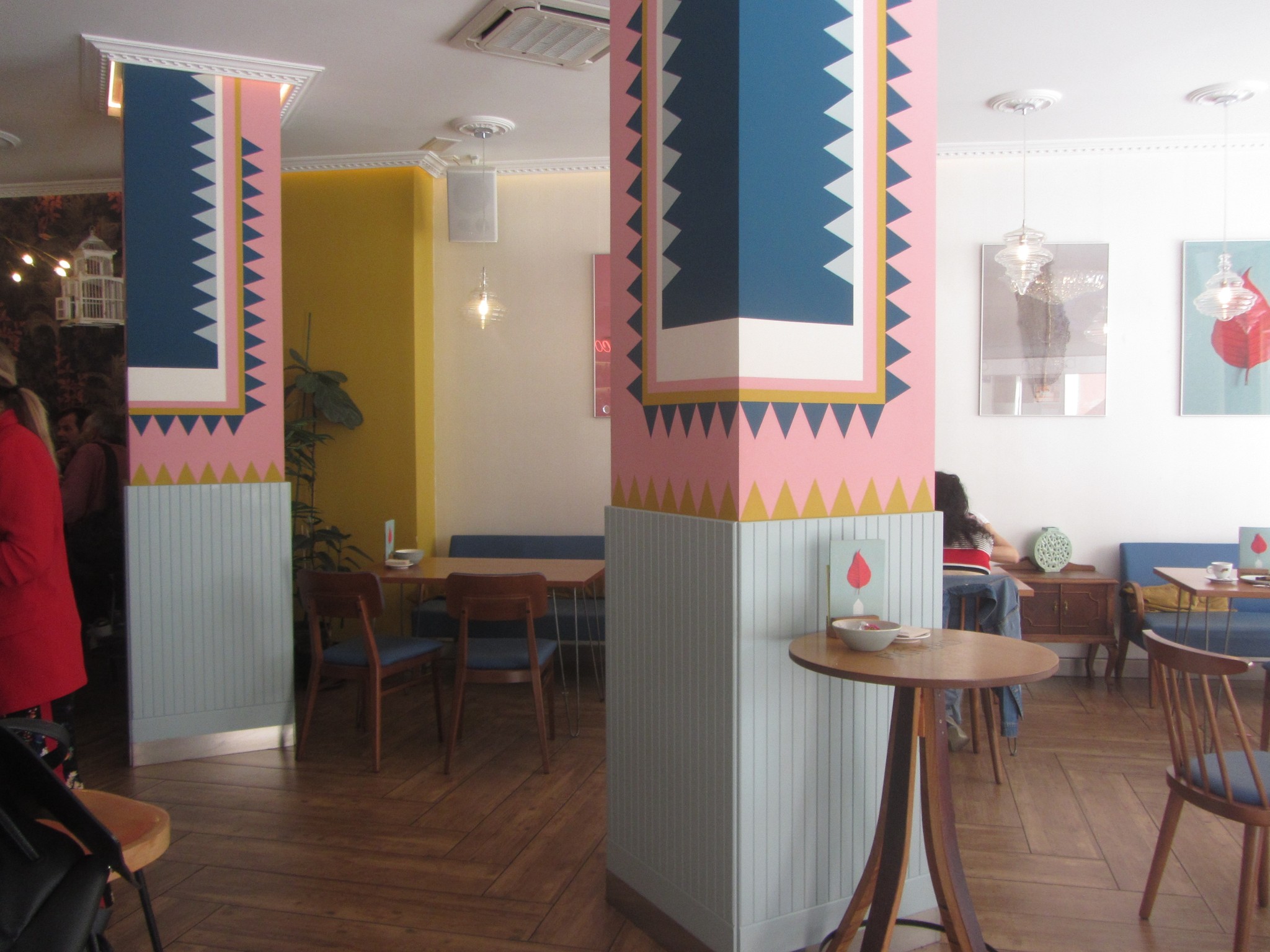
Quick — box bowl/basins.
[396,549,423,564]
[831,619,902,652]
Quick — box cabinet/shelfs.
[991,562,1122,695]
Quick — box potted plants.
[288,307,367,683]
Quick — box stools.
[45,789,173,952]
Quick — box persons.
[0,345,115,950]
[18,330,128,670]
[935,471,1020,749]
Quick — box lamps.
[458,115,516,331]
[1187,79,1259,321]
[982,88,1063,295]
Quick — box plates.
[386,562,414,570]
[1239,575,1270,586]
[892,633,931,644]
[1204,576,1239,583]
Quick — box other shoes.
[945,714,970,751]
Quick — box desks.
[990,565,1034,596]
[943,575,1023,785]
[355,558,604,737]
[1154,569,1270,757]
[786,625,1059,951]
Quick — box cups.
[1206,562,1233,579]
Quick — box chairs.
[1141,628,1270,952]
[434,573,559,775]
[298,566,444,770]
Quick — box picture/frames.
[979,244,1109,418]
[1180,235,1270,418]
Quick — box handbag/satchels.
[0,719,143,951]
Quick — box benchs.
[412,534,604,643]
[1117,540,1269,708]
